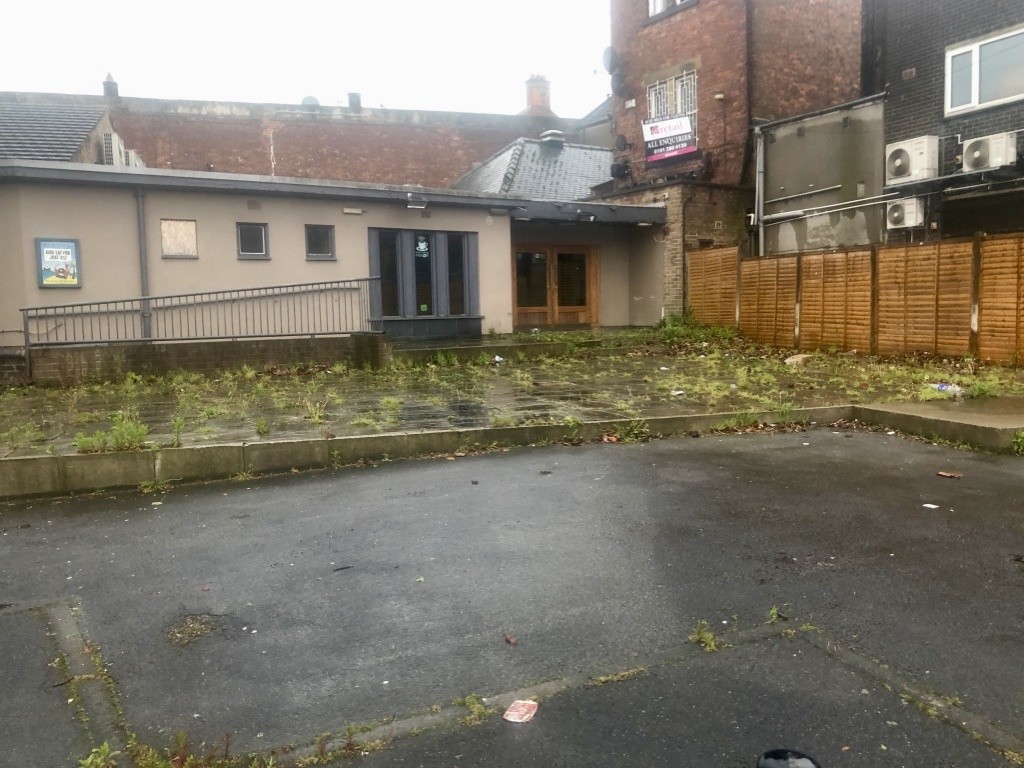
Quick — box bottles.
[929,383,951,391]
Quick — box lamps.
[404,200,430,209]
[714,92,724,100]
[577,211,596,224]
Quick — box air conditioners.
[885,134,939,187]
[963,131,1017,174]
[886,196,925,232]
